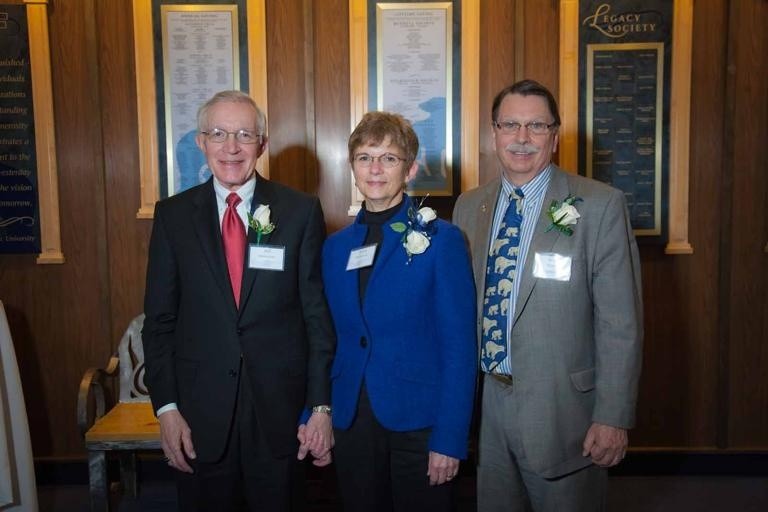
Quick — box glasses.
[494,117,557,133]
[200,128,263,144]
[352,153,409,167]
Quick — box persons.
[451,79,647,511]
[298,110,478,511]
[140,89,337,511]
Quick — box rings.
[163,456,170,463]
[447,472,454,479]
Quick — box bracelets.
[312,406,334,416]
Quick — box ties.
[222,192,248,311]
[480,187,525,378]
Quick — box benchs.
[74,314,174,510]
